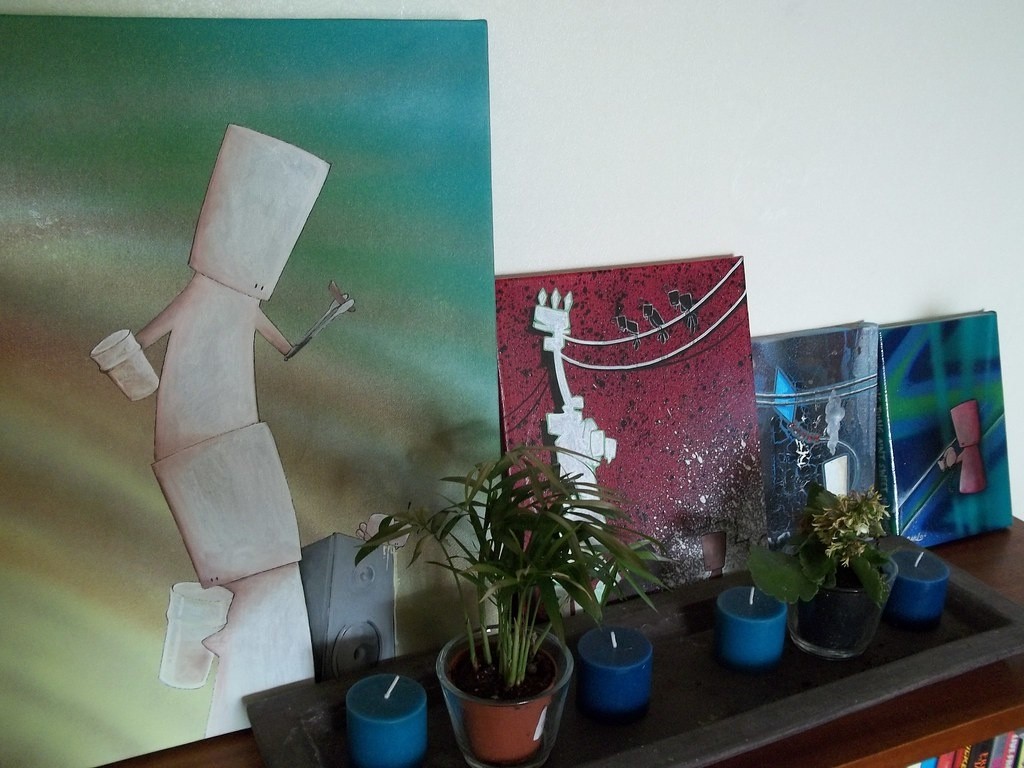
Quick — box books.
[907,728,1024,768]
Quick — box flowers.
[747,482,930,611]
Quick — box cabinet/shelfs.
[98,517,1024,768]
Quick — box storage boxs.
[495,254,768,614]
[754,320,880,550]
[875,310,1014,548]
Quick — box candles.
[578,625,654,727]
[714,586,787,674]
[347,673,428,768]
[885,548,951,632]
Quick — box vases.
[799,558,894,650]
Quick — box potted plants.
[348,444,682,762]
